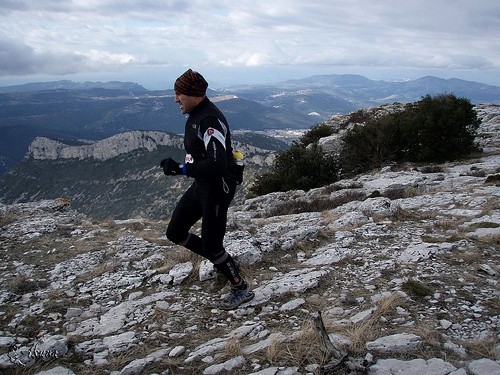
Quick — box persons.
[158,68,255,311]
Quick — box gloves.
[160,158,183,177]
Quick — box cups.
[233,150,245,167]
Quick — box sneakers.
[218,281,255,310]
[210,272,228,293]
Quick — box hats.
[174,69,208,98]
